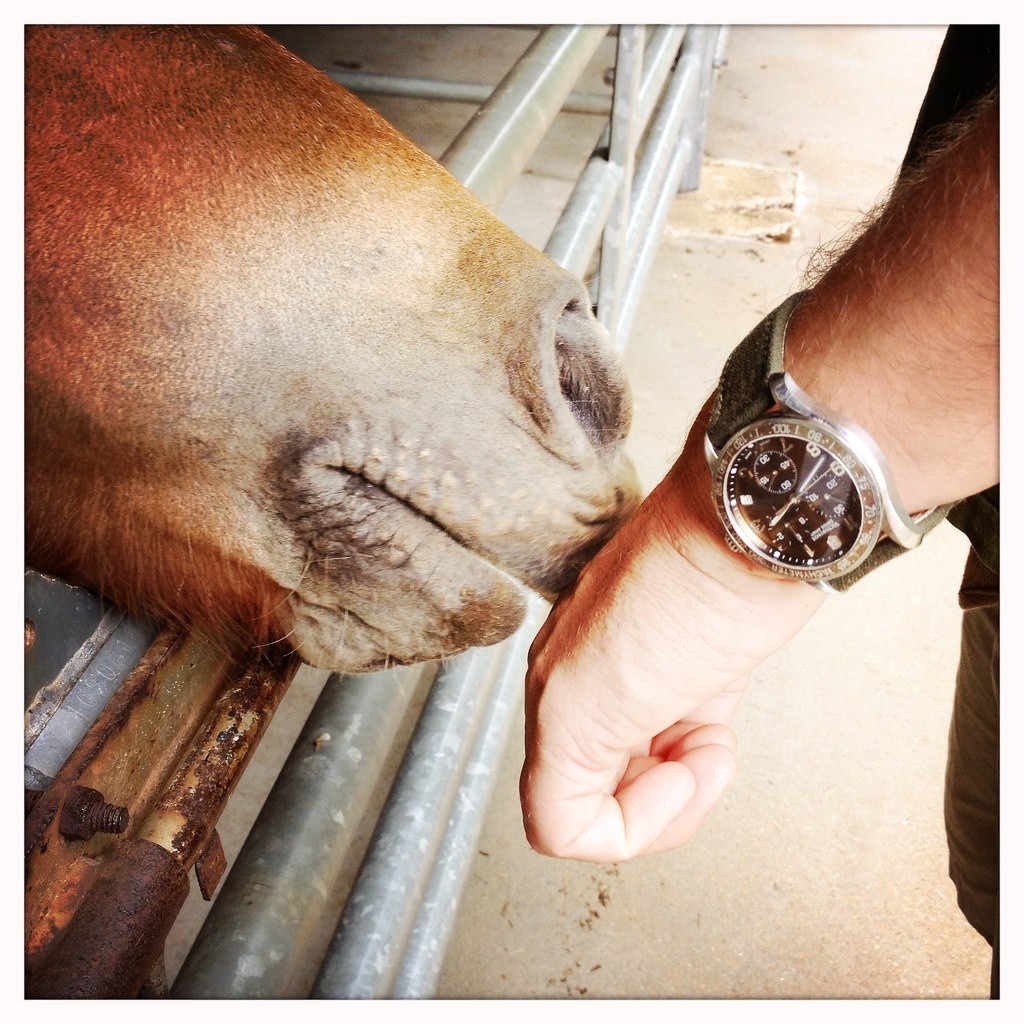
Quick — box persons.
[518,25,1000,1000]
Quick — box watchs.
[704,289,955,598]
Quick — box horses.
[26,28,644,680]
